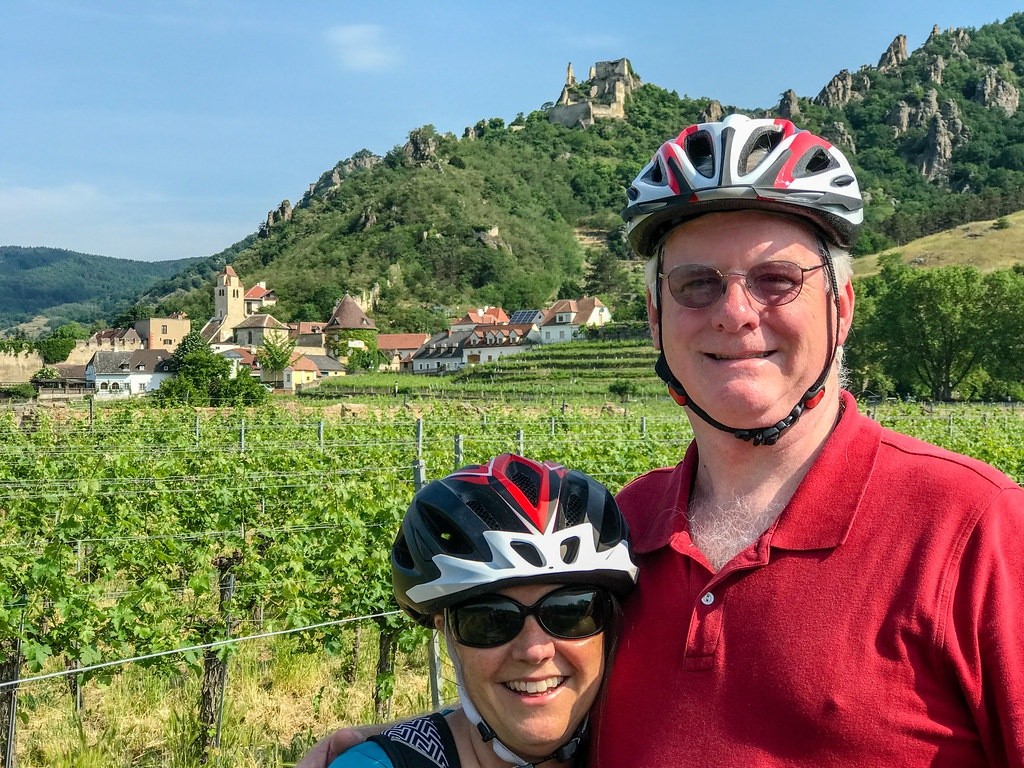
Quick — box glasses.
[444,582,615,649]
[657,260,832,309]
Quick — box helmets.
[390,452,640,629]
[621,113,865,258]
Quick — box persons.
[319,452,641,768]
[297,115,1024,768]
